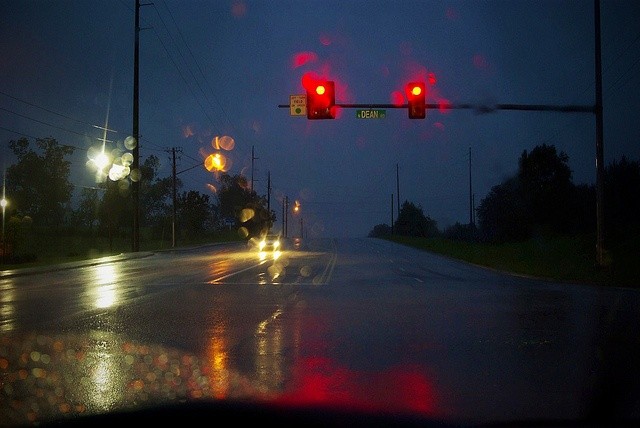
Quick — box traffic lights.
[408,82,425,119]
[307,81,334,119]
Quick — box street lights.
[172,158,221,246]
[285,196,298,237]
[107,169,127,253]
[0,199,7,261]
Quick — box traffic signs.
[356,110,386,119]
